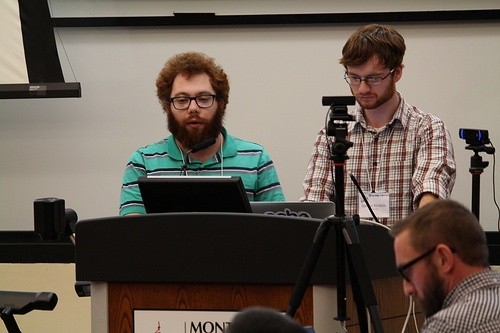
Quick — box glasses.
[169,95,218,110]
[397,246,456,283]
[344,67,398,85]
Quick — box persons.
[301,24,456,228]
[390,199,500,333]
[120,52,288,216]
[225,309,311,333]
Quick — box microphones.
[184,138,216,176]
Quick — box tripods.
[286,131,385,333]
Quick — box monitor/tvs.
[136,175,253,214]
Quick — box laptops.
[249,201,337,218]
